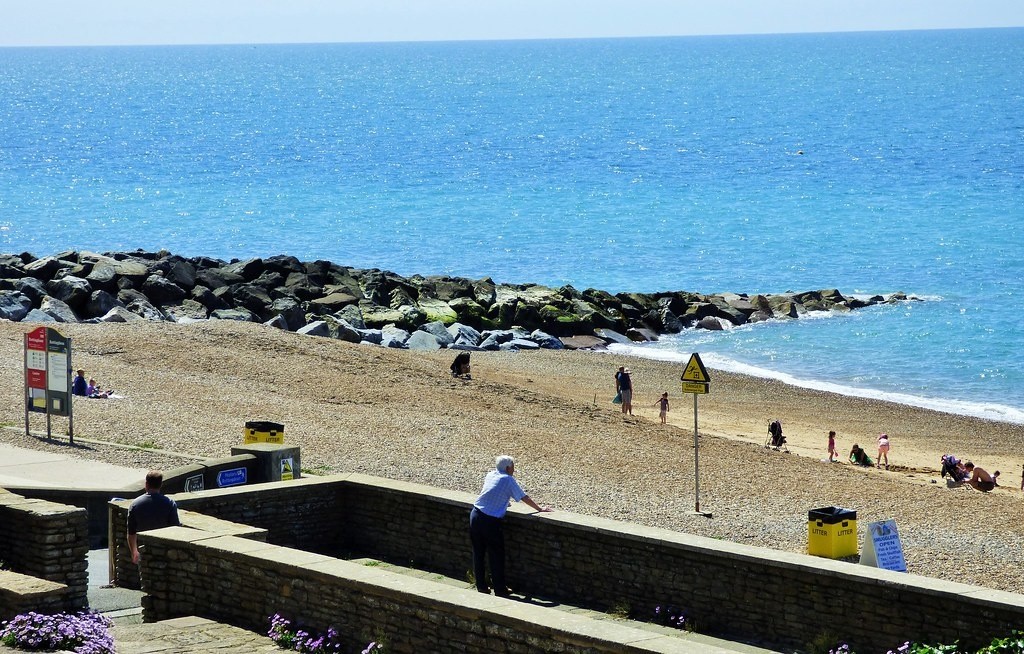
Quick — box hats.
[624,368,631,374]
[662,392,668,396]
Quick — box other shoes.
[495,589,513,596]
[479,587,491,594]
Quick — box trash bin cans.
[246,421,285,445]
[808,505,858,560]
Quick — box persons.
[469,455,554,596]
[127,472,180,564]
[450,350,470,373]
[86,379,114,395]
[964,462,994,493]
[828,431,838,462]
[849,444,874,467]
[654,392,669,424]
[73,369,100,396]
[878,434,889,469]
[615,367,633,414]
[990,471,1000,487]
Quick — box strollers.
[765,419,790,453]
[450,349,472,380]
[941,454,974,491]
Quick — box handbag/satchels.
[612,392,622,404]
